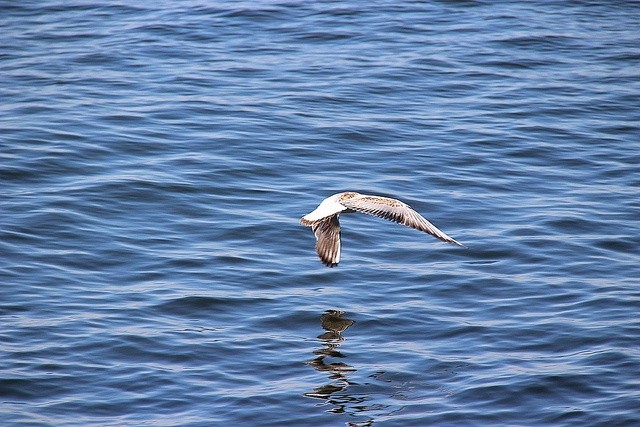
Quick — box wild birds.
[300,191,468,268]
